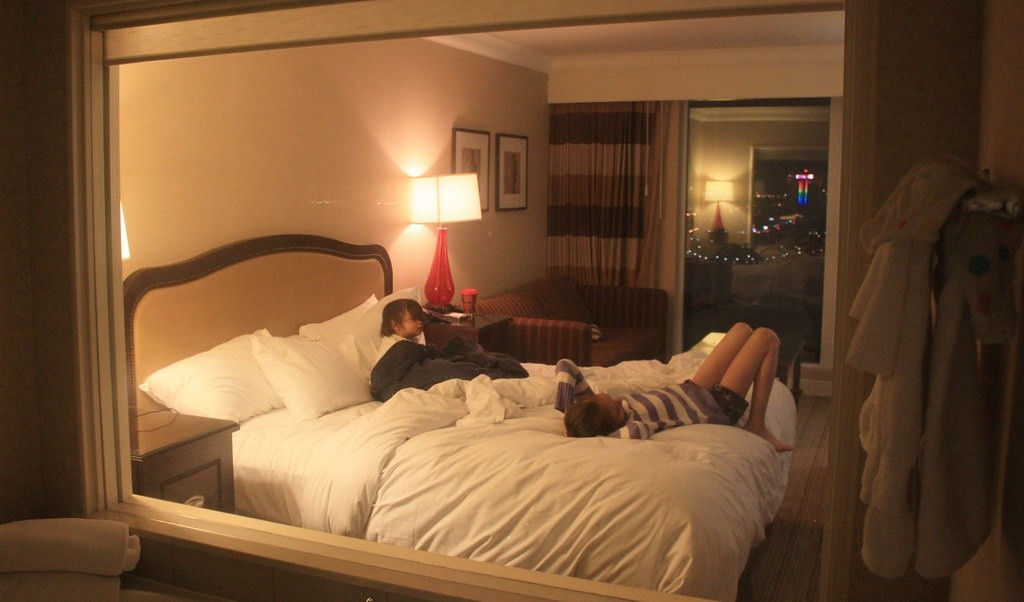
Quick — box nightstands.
[129,407,241,514]
[423,313,513,355]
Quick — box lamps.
[703,181,737,244]
[409,172,482,308]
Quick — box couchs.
[455,280,668,368]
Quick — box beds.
[122,233,797,602]
[729,243,815,305]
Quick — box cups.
[460,289,479,314]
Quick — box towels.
[848,154,1018,579]
[0,518,142,602]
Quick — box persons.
[553,323,795,452]
[370,298,449,395]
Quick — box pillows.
[138,282,425,426]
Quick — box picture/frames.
[452,127,491,212]
[495,133,529,211]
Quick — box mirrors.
[682,96,831,365]
